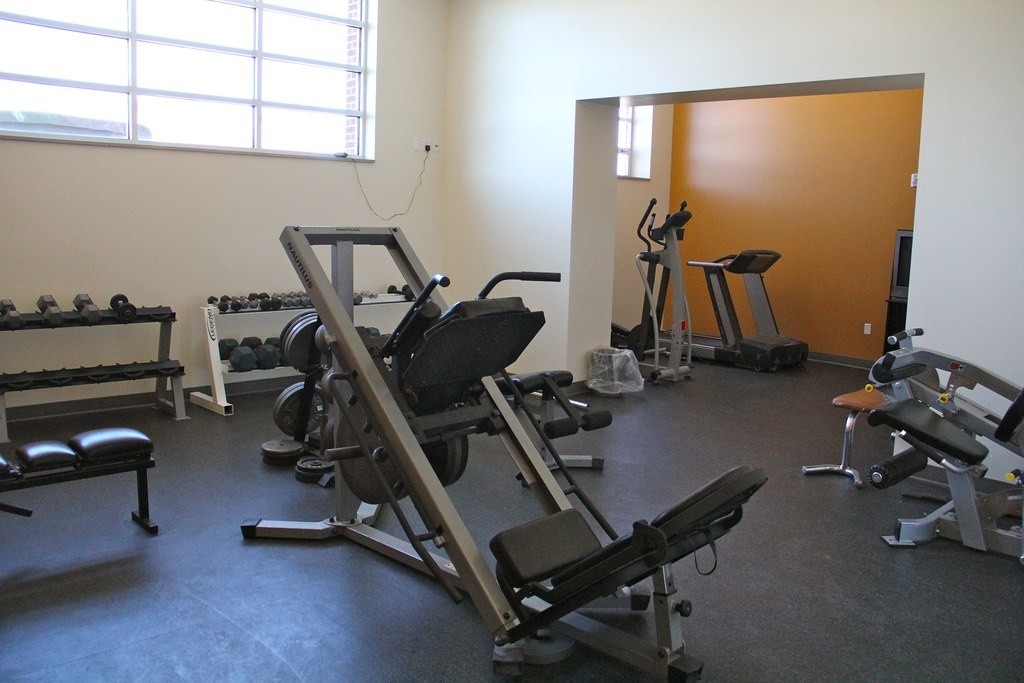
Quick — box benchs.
[0,427,158,536]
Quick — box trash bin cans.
[590,348,627,398]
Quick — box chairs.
[487,464,770,644]
